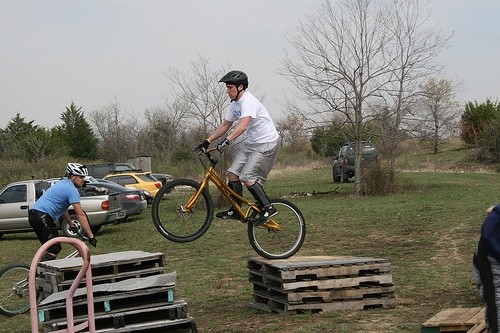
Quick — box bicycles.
[151,143,307,260]
[0,225,96,317]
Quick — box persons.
[28,162,97,280]
[471,201,500,333]
[193,70,282,226]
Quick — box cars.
[101,172,163,204]
[50,176,148,224]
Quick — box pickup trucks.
[0,178,112,237]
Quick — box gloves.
[89,237,97,246]
[217,138,229,153]
[194,138,209,153]
[70,225,78,232]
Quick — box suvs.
[84,163,175,193]
[332,140,381,184]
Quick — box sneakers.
[216,207,240,220]
[256,205,278,225]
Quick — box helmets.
[66,162,89,176]
[218,70,248,90]
